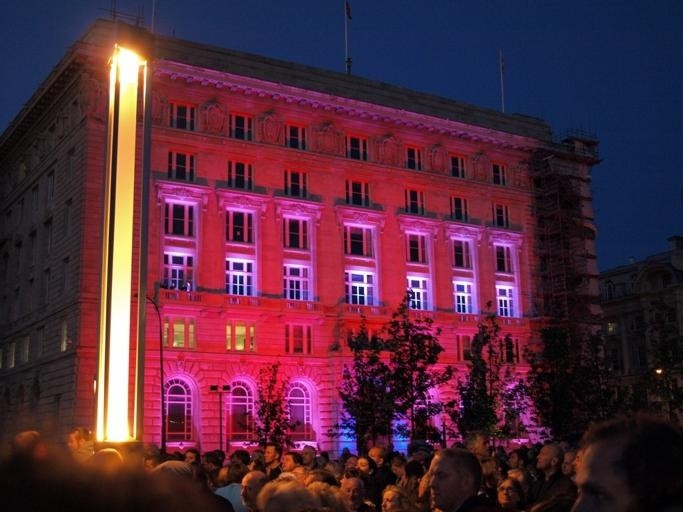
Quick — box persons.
[1,417,682,512]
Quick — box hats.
[416,446,431,456]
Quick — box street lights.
[209,384,232,450]
[133,292,167,453]
[655,364,669,425]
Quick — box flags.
[500,55,506,75]
[346,2,354,20]
